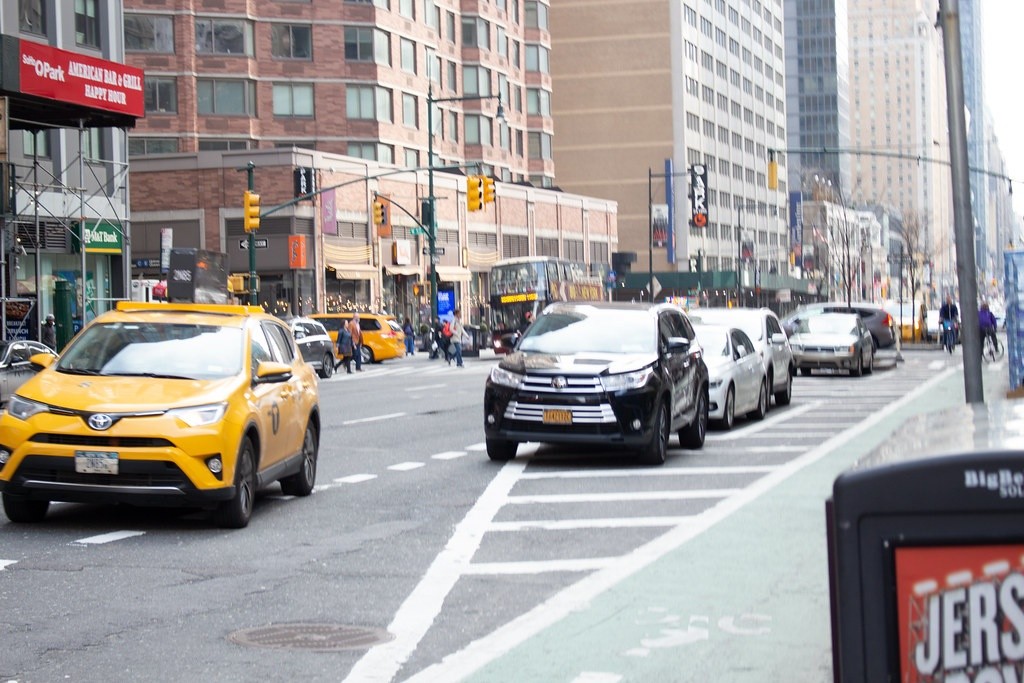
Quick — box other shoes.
[347,371,353,374]
[334,366,337,372]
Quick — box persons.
[939,298,959,346]
[978,303,999,354]
[403,317,415,355]
[429,310,470,367]
[334,313,363,373]
[41,314,56,350]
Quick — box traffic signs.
[239,239,268,249]
[423,248,444,255]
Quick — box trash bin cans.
[461,324,481,356]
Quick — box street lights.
[428,85,505,330]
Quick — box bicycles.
[940,319,959,354]
[982,317,1004,364]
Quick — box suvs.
[688,307,796,410]
[307,314,405,364]
[779,304,896,353]
[483,303,708,465]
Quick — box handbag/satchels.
[443,322,456,336]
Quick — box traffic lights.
[467,177,482,211]
[373,202,384,225]
[227,274,250,295]
[483,176,496,203]
[243,191,260,233]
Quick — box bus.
[480,256,607,357]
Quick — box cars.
[0,340,60,404]
[688,325,766,428]
[279,316,334,378]
[789,312,875,377]
[0,304,321,530]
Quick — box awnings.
[329,265,379,279]
[436,266,471,281]
[385,266,422,275]
